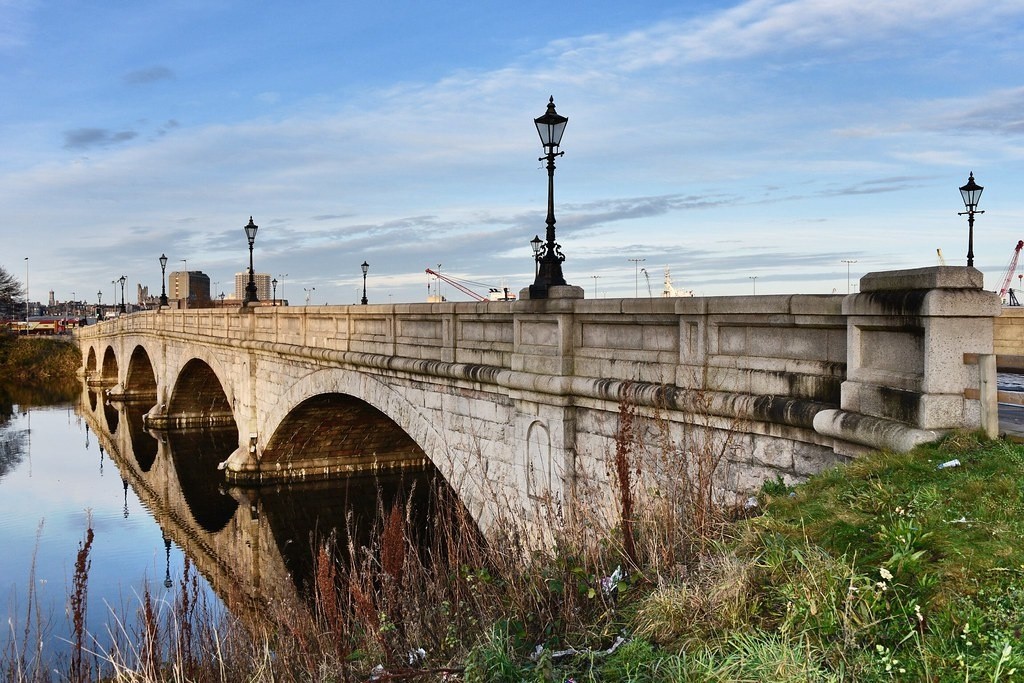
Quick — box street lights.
[119,275,126,315]
[530,234,544,279]
[360,261,370,305]
[850,283,858,293]
[180,259,187,309]
[23,257,30,336]
[220,291,226,308]
[528,94,568,300]
[97,290,102,305]
[271,278,278,306]
[277,273,289,300]
[303,288,316,305]
[213,281,220,298]
[957,171,986,267]
[839,259,858,294]
[158,253,169,309]
[628,258,646,298]
[242,215,261,307]
[72,292,76,317]
[590,275,602,298]
[749,276,759,295]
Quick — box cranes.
[993,240,1024,298]
[423,268,491,302]
